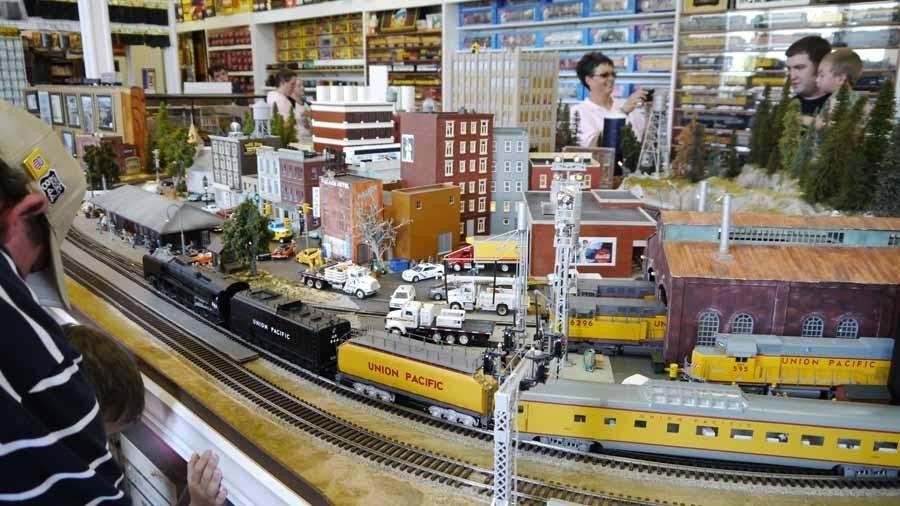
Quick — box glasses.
[591,71,616,79]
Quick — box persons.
[790,47,864,133]
[784,33,834,116]
[54,318,229,506]
[0,96,130,506]
[576,240,592,264]
[206,64,248,124]
[287,77,312,136]
[262,67,298,122]
[565,52,651,152]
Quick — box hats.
[0,102,88,316]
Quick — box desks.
[0,248,899,505]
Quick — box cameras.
[643,88,655,102]
